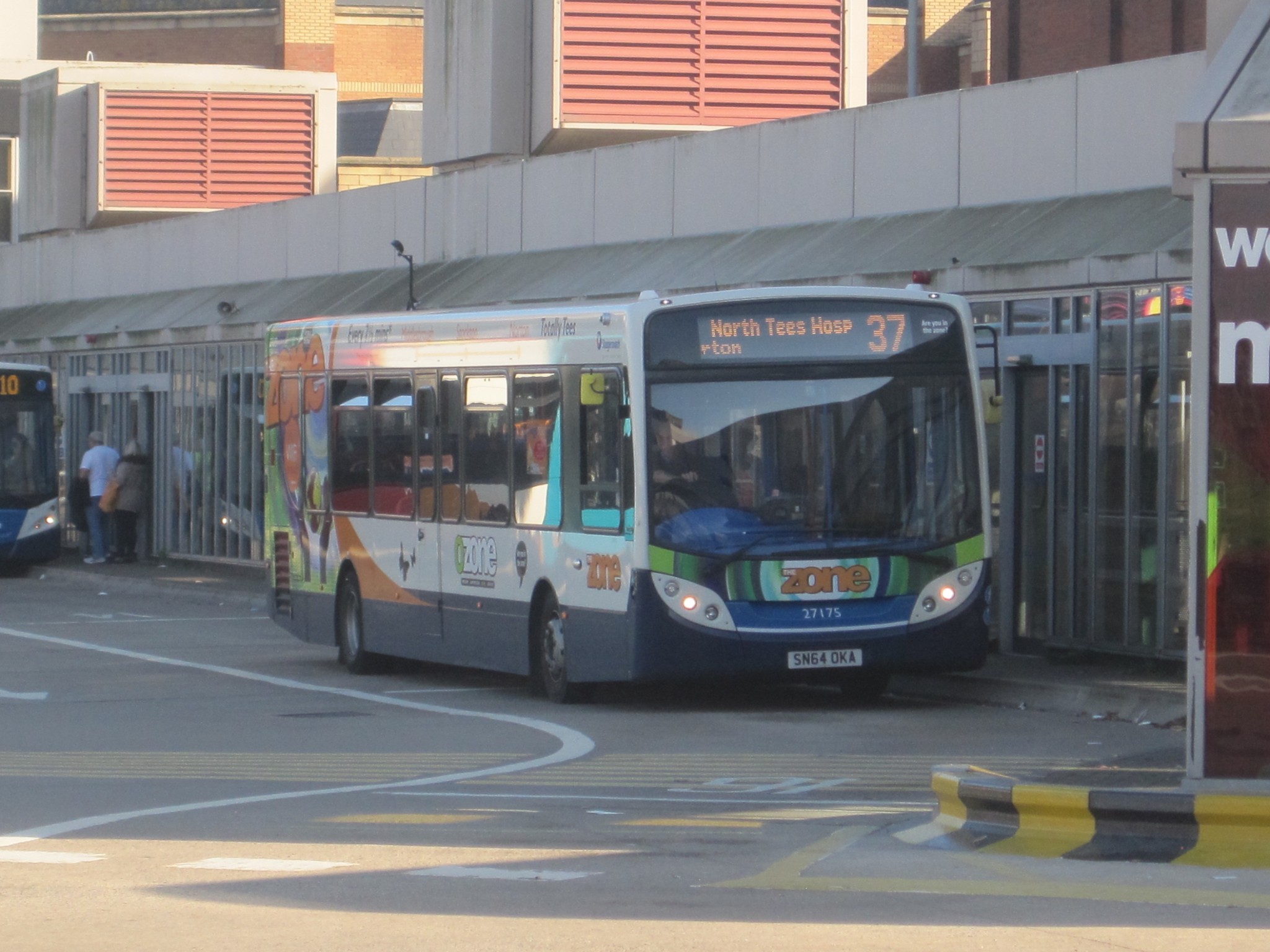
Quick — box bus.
[258,285,1003,706]
[0,363,64,568]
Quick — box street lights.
[390,238,414,309]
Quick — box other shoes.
[107,552,138,563]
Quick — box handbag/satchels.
[99,479,121,513]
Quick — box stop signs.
[1034,435,1045,474]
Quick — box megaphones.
[217,299,237,317]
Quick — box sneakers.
[84,556,106,564]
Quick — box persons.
[98,439,148,563]
[172,433,193,493]
[77,430,121,564]
[649,420,710,514]
[3,435,37,494]
